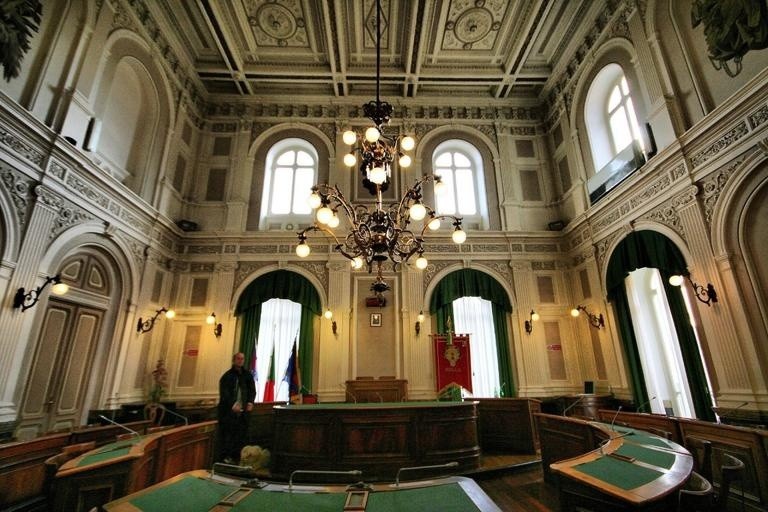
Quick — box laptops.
[578,381,595,396]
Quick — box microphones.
[375,392,384,403]
[285,469,361,490]
[392,462,459,488]
[609,406,623,432]
[494,382,507,398]
[158,405,188,426]
[718,402,748,426]
[338,384,357,404]
[436,385,463,402]
[597,431,635,455]
[208,462,252,480]
[636,397,656,413]
[98,414,141,442]
[563,395,585,418]
[400,393,427,402]
[301,385,321,405]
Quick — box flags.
[250,336,259,383]
[263,345,275,402]
[280,338,300,403]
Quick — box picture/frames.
[369,312,382,327]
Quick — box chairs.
[678,434,750,512]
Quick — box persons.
[215,352,258,472]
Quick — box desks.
[1,393,696,512]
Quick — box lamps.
[136,307,223,339]
[666,271,717,311]
[414,310,425,337]
[523,303,606,336]
[12,272,70,313]
[294,1,468,300]
[325,308,337,335]
